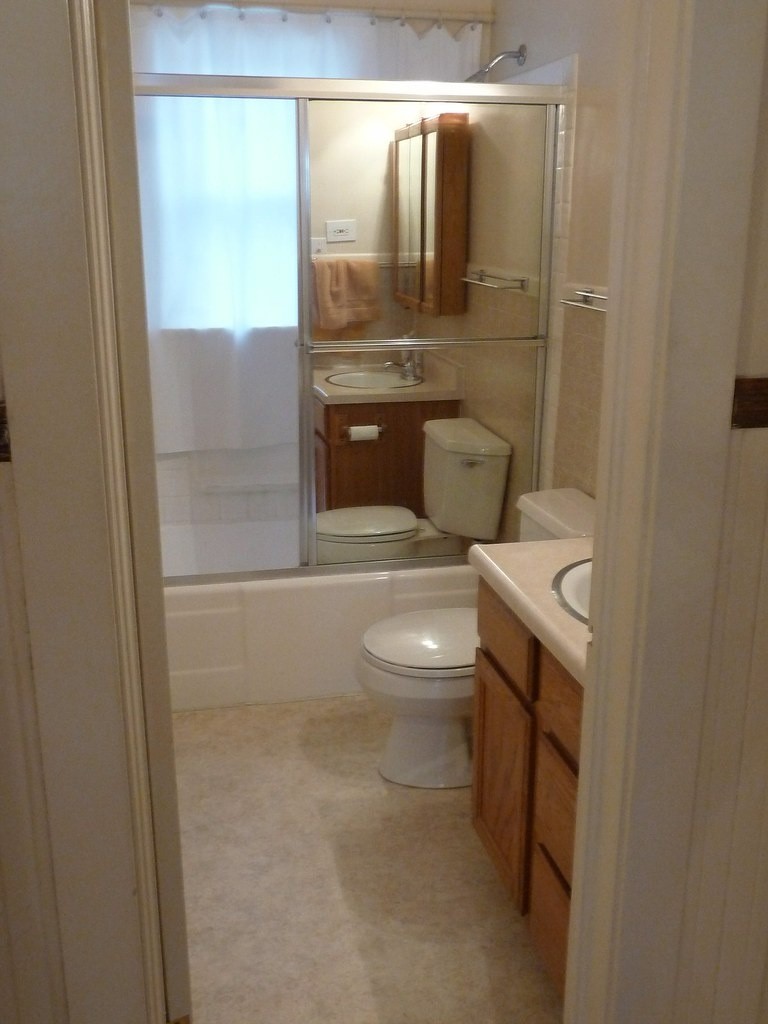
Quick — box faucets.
[383,334,423,382]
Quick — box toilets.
[356,487,598,789]
[315,417,514,567]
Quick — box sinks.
[550,558,592,625]
[324,370,426,389]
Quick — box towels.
[311,259,386,360]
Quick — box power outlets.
[327,219,357,242]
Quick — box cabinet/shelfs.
[390,113,474,316]
[467,578,585,971]
[313,396,461,521]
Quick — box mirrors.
[308,100,559,567]
[395,128,437,304]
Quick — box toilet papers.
[348,424,379,442]
